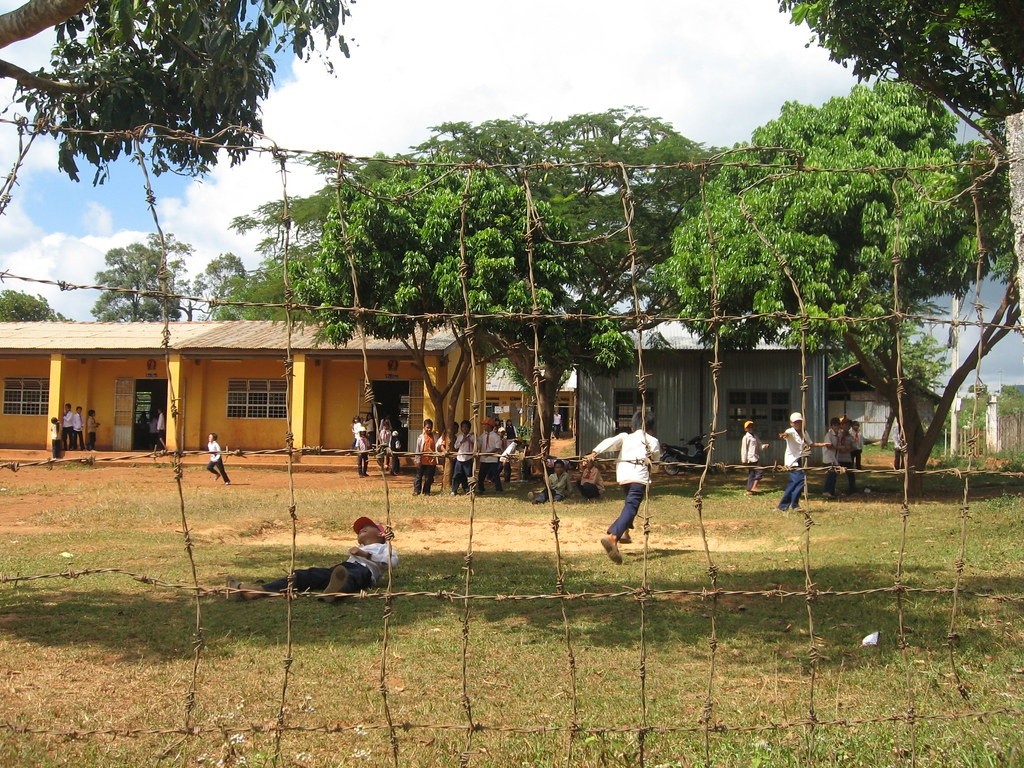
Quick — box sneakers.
[601,534,622,563]
[619,531,632,543]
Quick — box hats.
[358,427,367,432]
[842,419,852,425]
[498,427,506,432]
[514,437,523,442]
[480,418,496,428]
[790,411,803,422]
[353,517,387,540]
[392,431,399,436]
[743,421,753,428]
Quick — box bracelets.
[367,550,374,560]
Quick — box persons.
[62,402,74,451]
[72,406,85,451]
[526,459,572,505]
[822,416,864,499]
[346,412,520,497]
[50,417,62,459]
[582,410,661,565]
[225,517,399,603]
[553,408,562,440]
[577,454,606,500]
[740,420,770,495]
[149,407,168,458]
[206,432,230,485]
[775,413,832,512]
[87,409,101,451]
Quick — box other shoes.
[392,471,399,476]
[214,473,220,481]
[412,488,505,497]
[746,489,763,495]
[383,464,393,469]
[225,480,230,485]
[345,452,353,456]
[822,493,835,498]
[359,474,369,478]
[794,507,804,512]
[317,565,349,601]
[226,578,244,601]
[528,492,538,504]
[579,496,590,503]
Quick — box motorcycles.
[659,431,715,476]
